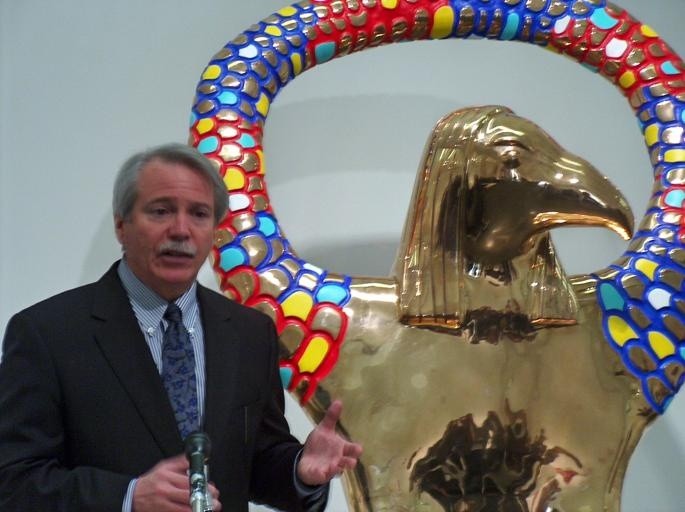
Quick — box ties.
[160,303,200,441]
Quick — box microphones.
[183,430,213,512]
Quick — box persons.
[1,143,363,512]
